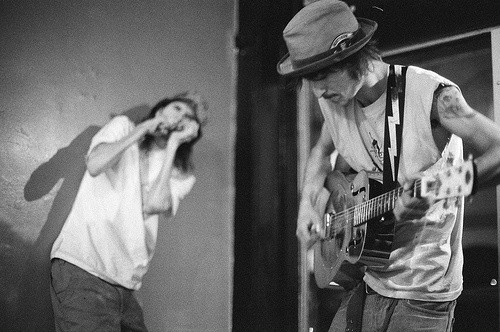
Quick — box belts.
[345,280,377,332]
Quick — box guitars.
[312,158,476,292]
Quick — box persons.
[49,90,209,332]
[277,0,500,332]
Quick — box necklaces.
[354,95,383,172]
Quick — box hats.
[277,0,378,77]
